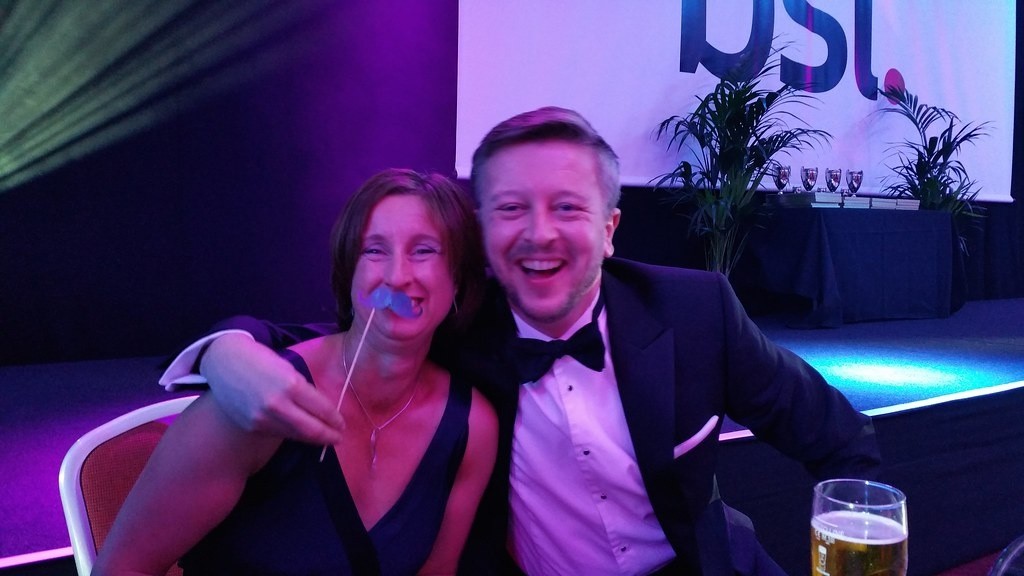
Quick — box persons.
[159,106,885,576]
[86,166,501,576]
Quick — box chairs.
[59,395,200,576]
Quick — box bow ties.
[506,285,606,385]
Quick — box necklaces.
[342,332,418,468]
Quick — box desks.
[754,208,953,328]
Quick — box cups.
[809,478,910,576]
